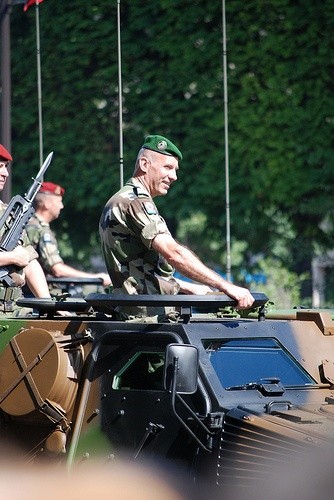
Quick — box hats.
[39,181,64,196]
[0,144,12,161]
[143,134,182,161]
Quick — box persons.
[25,178,112,297]
[0,142,58,316]
[0,425,191,499]
[99,134,255,313]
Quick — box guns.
[0,149,54,288]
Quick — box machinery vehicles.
[1,280,333,500]
